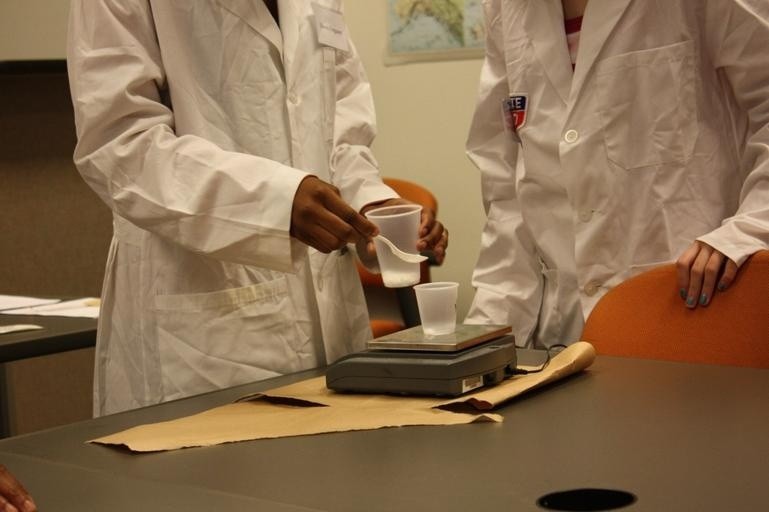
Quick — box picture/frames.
[380,1,487,67]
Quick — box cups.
[413,281,460,335]
[364,204,423,288]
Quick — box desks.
[0,296,107,440]
[0,348,769,512]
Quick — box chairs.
[580,251,769,370]
[354,177,445,340]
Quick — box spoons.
[374,236,429,265]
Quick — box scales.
[326,324,518,397]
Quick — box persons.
[65,0,451,418]
[1,462,38,512]
[463,1,769,350]
